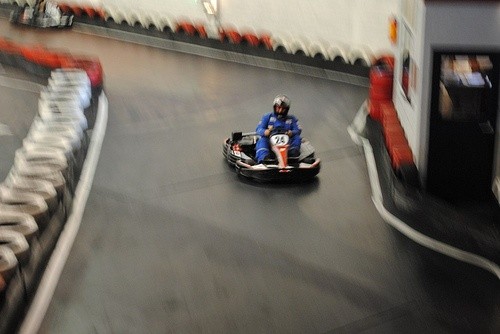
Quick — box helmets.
[273,94,290,118]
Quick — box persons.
[255,95,301,163]
